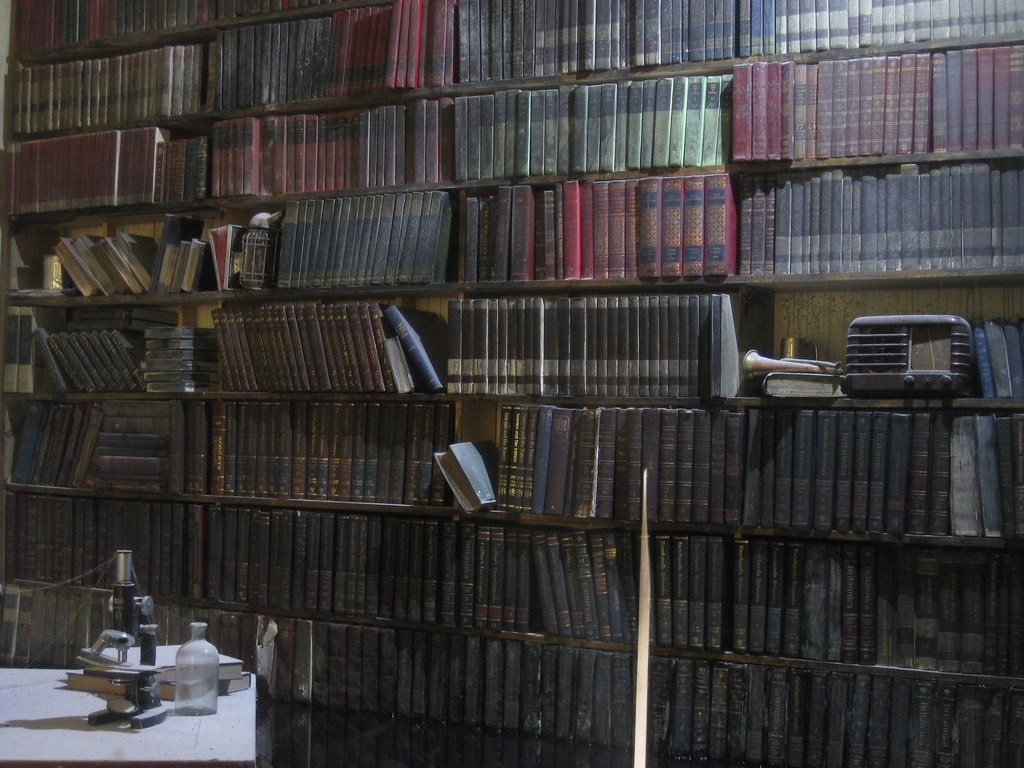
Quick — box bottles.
[175,622,219,715]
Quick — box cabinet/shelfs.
[0,0,1024,768]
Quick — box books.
[0,0,1024,768]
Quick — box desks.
[0,669,256,768]
